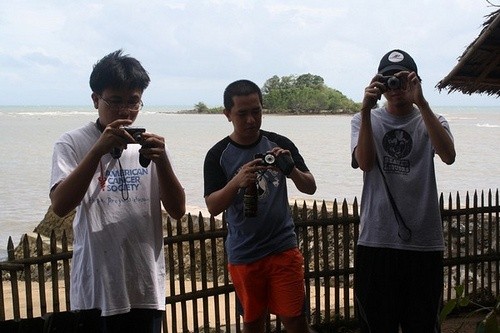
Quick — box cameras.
[254,152,278,167]
[121,127,146,144]
[378,76,401,89]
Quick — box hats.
[378,50,418,75]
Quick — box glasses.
[93,91,144,112]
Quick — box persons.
[205,79,316,333]
[351,50,456,333]
[46,50,184,333]
